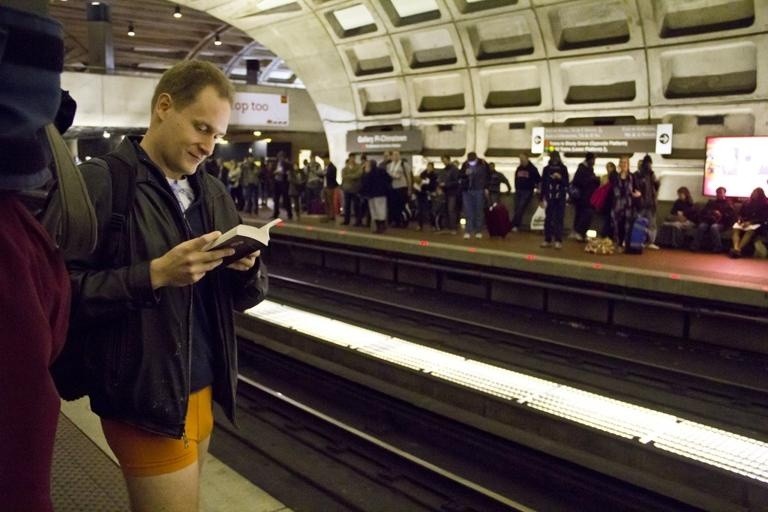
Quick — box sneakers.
[555,241,562,248]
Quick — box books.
[200,217,283,269]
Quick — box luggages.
[487,195,513,239]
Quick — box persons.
[341,150,414,233]
[42,59,271,512]
[541,151,570,247]
[730,188,767,257]
[664,187,696,247]
[692,188,735,252]
[0,192,71,512]
[417,152,510,239]
[205,152,337,222]
[568,152,598,240]
[606,155,660,256]
[511,152,542,231]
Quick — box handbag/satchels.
[565,183,578,206]
[590,183,610,212]
[630,216,649,252]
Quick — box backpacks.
[50,155,128,404]
[545,173,563,205]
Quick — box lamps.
[126,6,223,47]
